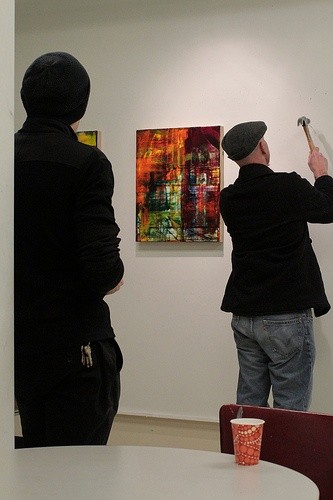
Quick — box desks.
[0,445,319,500]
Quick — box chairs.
[219,405,333,500]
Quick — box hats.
[21,51,90,125]
[221,121,267,161]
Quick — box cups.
[230,418,265,466]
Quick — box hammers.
[297,116,314,153]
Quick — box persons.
[219,121,333,412]
[13,53,126,446]
